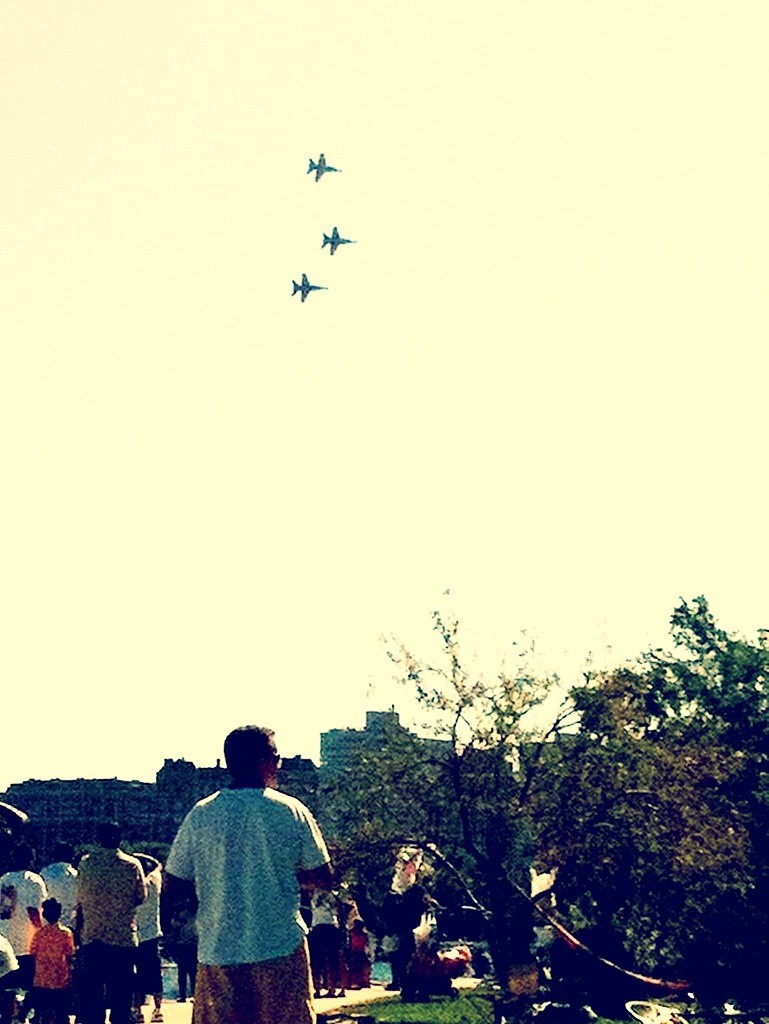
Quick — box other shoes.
[150,1010,164,1022]
[135,1012,145,1023]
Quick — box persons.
[0,822,162,1024]
[436,942,494,978]
[383,881,437,992]
[164,725,334,1024]
[309,879,372,999]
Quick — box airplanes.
[305,153,343,183]
[320,227,358,256]
[290,273,329,303]
